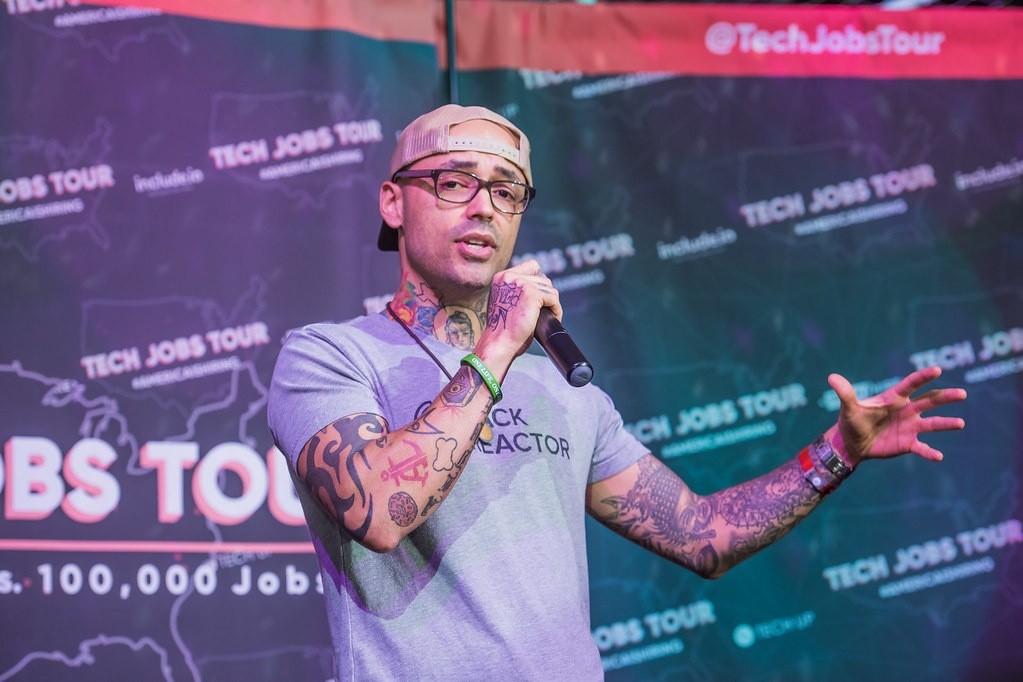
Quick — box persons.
[267,104,967,682]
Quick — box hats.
[376,103,533,251]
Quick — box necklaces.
[385,301,494,443]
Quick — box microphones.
[533,305,594,388]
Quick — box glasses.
[391,169,536,214]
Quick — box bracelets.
[798,447,837,495]
[460,354,503,405]
[813,434,854,479]
